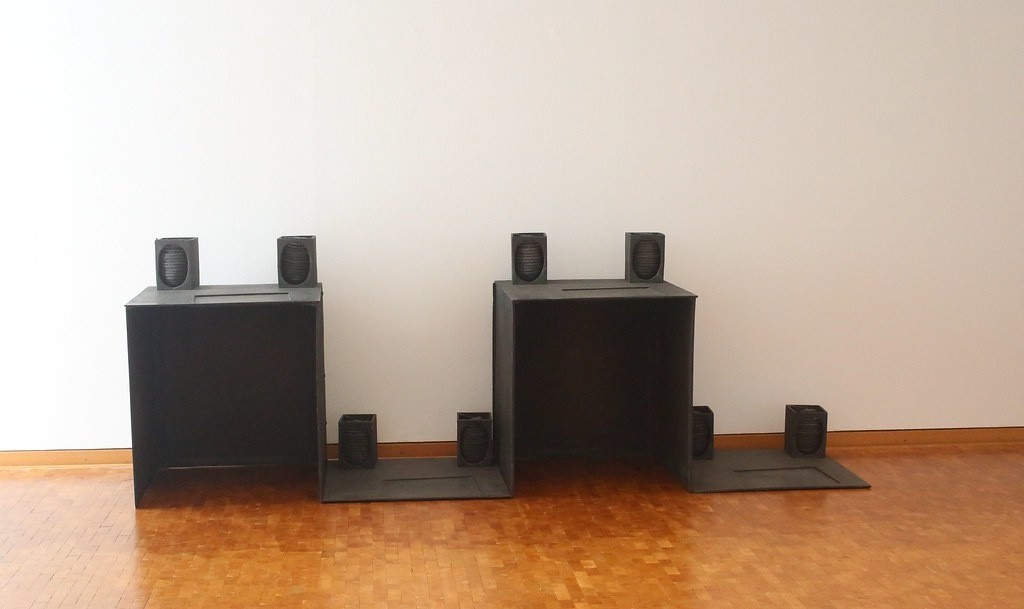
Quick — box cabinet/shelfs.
[125,284,327,508]
[493,281,698,495]
